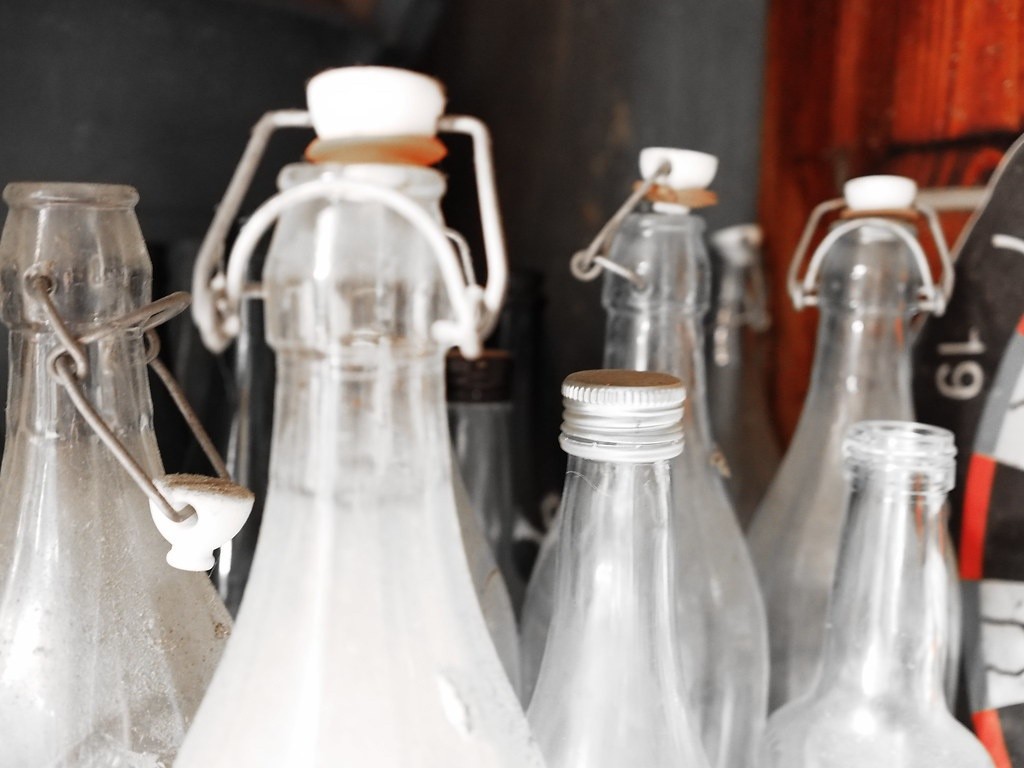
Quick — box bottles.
[0,59,1003,765]
